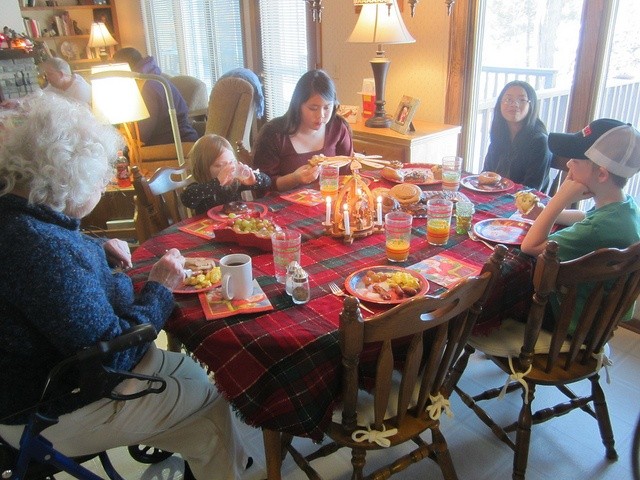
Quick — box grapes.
[228,211,278,238]
[363,269,389,286]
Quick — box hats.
[548,117,640,179]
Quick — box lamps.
[347,0,416,128]
[89,23,119,59]
[91,62,150,182]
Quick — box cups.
[426,198,453,247]
[318,164,340,201]
[441,155,463,191]
[455,201,474,234]
[270,230,302,284]
[229,162,251,181]
[384,211,413,263]
[218,253,254,301]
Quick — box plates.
[460,174,514,193]
[379,162,454,185]
[207,202,267,222]
[171,257,222,293]
[344,265,430,305]
[411,190,475,219]
[340,175,372,190]
[472,217,536,246]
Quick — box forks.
[467,230,494,252]
[328,281,375,315]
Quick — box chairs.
[276,243,509,480]
[130,165,196,228]
[449,243,640,479]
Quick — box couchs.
[169,76,209,118]
[130,76,257,183]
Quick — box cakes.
[431,164,446,179]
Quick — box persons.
[181,134,272,215]
[507,118,639,343]
[0,87,253,480]
[481,80,552,194]
[111,47,199,161]
[0,57,92,111]
[252,69,359,192]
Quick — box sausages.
[402,286,417,297]
[372,284,391,301]
[381,165,404,182]
[389,283,405,298]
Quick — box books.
[52,11,75,35]
[24,17,42,38]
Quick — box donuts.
[478,170,502,185]
[221,201,248,215]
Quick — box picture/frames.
[390,95,421,135]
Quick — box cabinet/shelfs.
[18,1,122,64]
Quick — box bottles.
[116,150,131,188]
[285,260,304,296]
[291,270,311,305]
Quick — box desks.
[348,112,463,163]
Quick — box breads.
[185,256,215,274]
[385,159,404,170]
[514,190,539,216]
[403,170,428,183]
[306,151,349,167]
[389,182,423,207]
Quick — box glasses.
[498,98,531,104]
